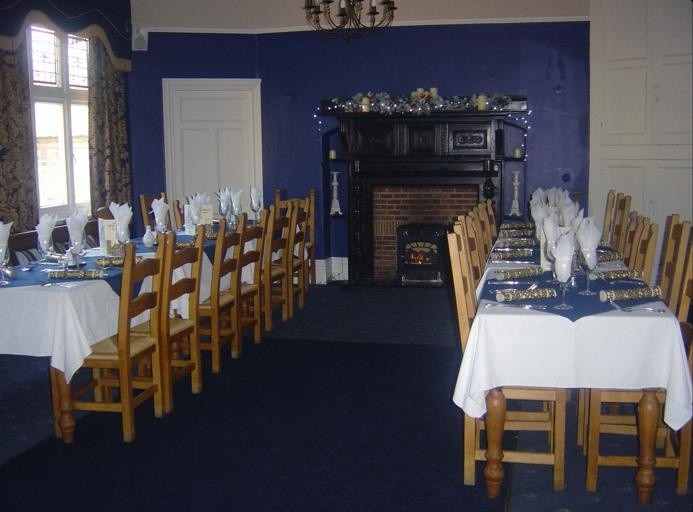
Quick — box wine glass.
[533,197,600,310]
[0,196,269,277]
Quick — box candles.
[430,86,439,101]
[476,95,487,110]
[415,87,424,102]
[514,147,521,160]
[329,149,338,160]
[362,95,371,111]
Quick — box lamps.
[301,1,399,39]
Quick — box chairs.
[583,189,692,480]
[446,192,570,492]
